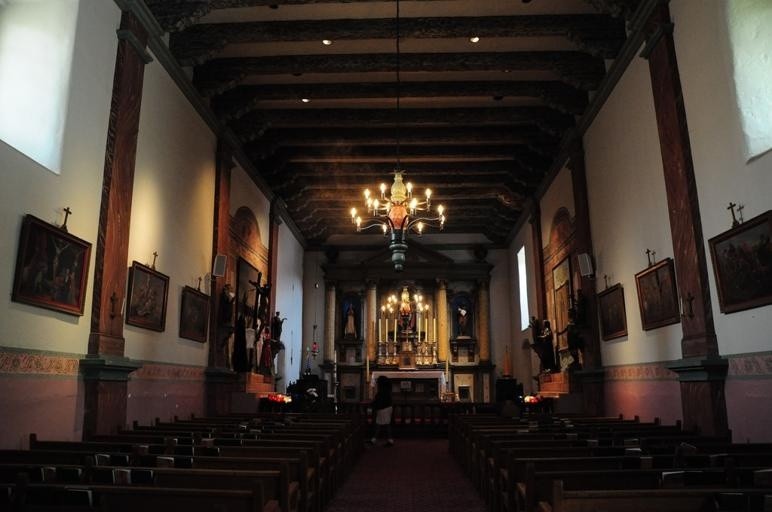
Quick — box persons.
[345,302,357,337]
[220,281,237,326]
[249,279,272,342]
[367,374,395,448]
[33,263,49,296]
[29,246,46,294]
[65,254,80,305]
[535,318,555,372]
[272,311,287,339]
[136,276,155,316]
[50,236,72,281]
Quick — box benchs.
[1,407,369,511]
[446,416,772,512]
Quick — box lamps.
[344,0,452,273]
[376,287,431,322]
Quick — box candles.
[416,312,439,342]
[376,314,397,344]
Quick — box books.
[22,412,304,511]
[510,410,772,511]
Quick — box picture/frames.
[11,215,92,317]
[633,248,682,331]
[178,282,210,343]
[707,206,771,317]
[125,260,170,332]
[597,274,628,343]
